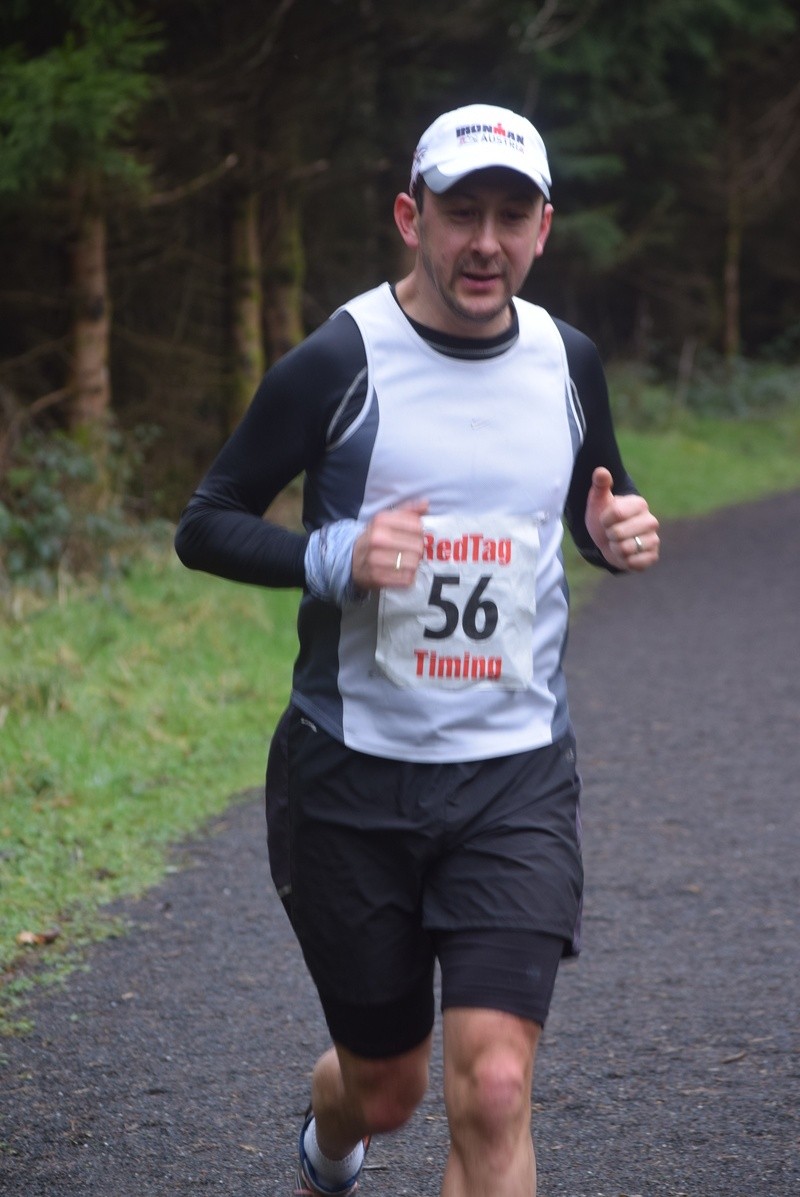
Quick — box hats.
[409,103,552,201]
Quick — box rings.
[395,550,402,571]
[633,536,642,552]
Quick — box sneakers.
[295,1102,370,1197]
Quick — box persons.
[174,103,660,1197]
